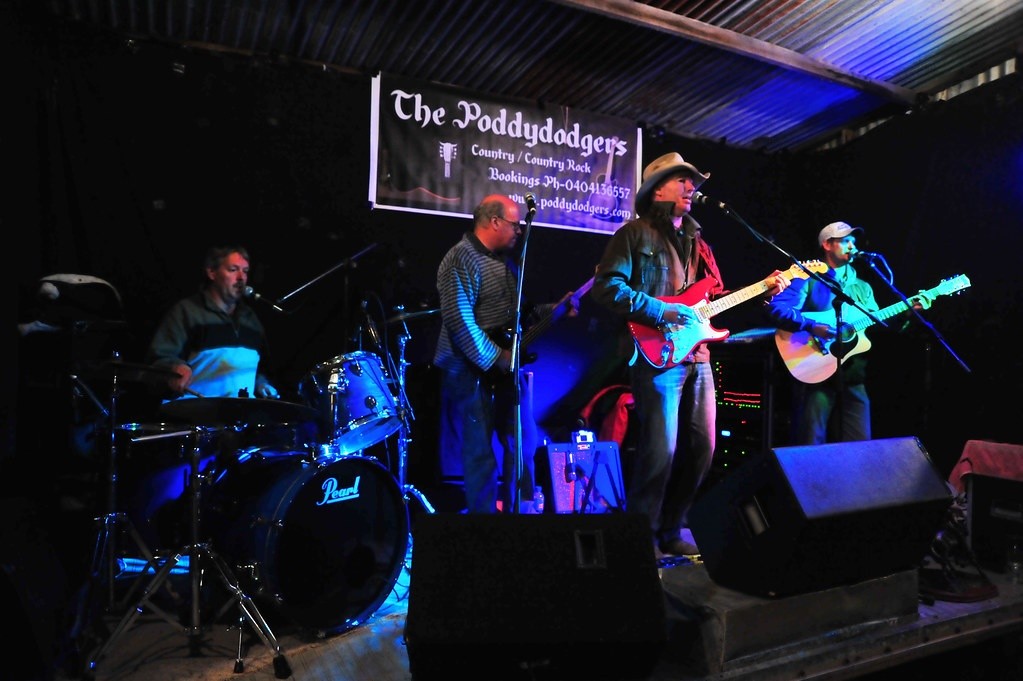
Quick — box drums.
[200,443,410,636]
[111,422,214,554]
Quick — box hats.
[635,152,710,216]
[818,221,864,247]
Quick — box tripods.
[66,364,293,681]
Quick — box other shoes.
[659,537,699,555]
[655,545,664,560]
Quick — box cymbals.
[144,397,323,425]
[375,308,442,326]
[78,358,184,382]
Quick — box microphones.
[691,191,731,210]
[240,286,284,314]
[360,298,383,348]
[849,249,880,258]
[524,192,536,215]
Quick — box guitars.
[627,258,831,373]
[477,262,599,390]
[773,272,972,388]
[295,350,404,457]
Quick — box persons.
[767,222,932,446]
[433,194,580,513]
[593,152,791,558]
[152,245,277,398]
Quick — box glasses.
[489,215,520,231]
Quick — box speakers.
[535,442,627,516]
[403,511,668,681]
[686,434,951,601]
